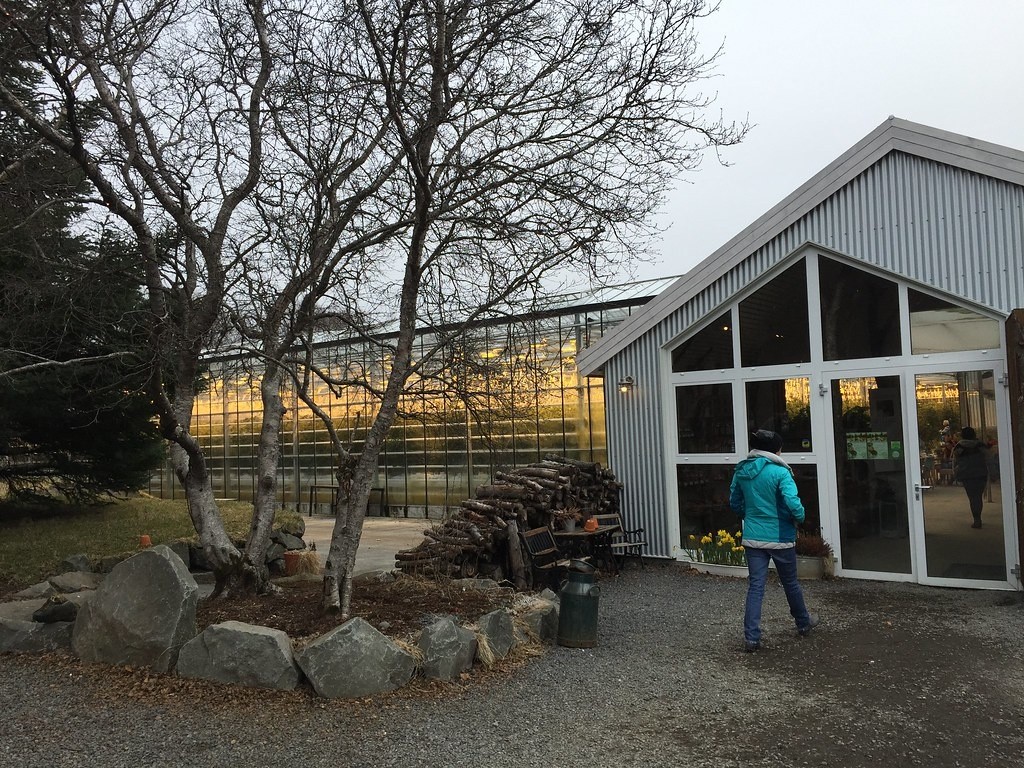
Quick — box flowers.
[670,530,749,567]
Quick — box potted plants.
[796,526,832,580]
[550,508,582,532]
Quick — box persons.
[729,429,820,652]
[847,442,877,458]
[939,420,992,528]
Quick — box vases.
[689,559,749,579]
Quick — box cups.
[590,518,598,528]
[585,520,595,531]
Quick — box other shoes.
[744,639,761,652]
[799,613,822,637]
[935,479,943,486]
[947,479,953,486]
[971,520,982,529]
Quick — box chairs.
[593,513,648,572]
[922,456,935,489]
[518,526,591,591]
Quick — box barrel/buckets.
[557,573,601,648]
[283,551,301,575]
[301,551,318,575]
[140,535,151,546]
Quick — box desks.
[552,525,620,576]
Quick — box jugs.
[564,518,575,531]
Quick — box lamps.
[616,375,637,392]
